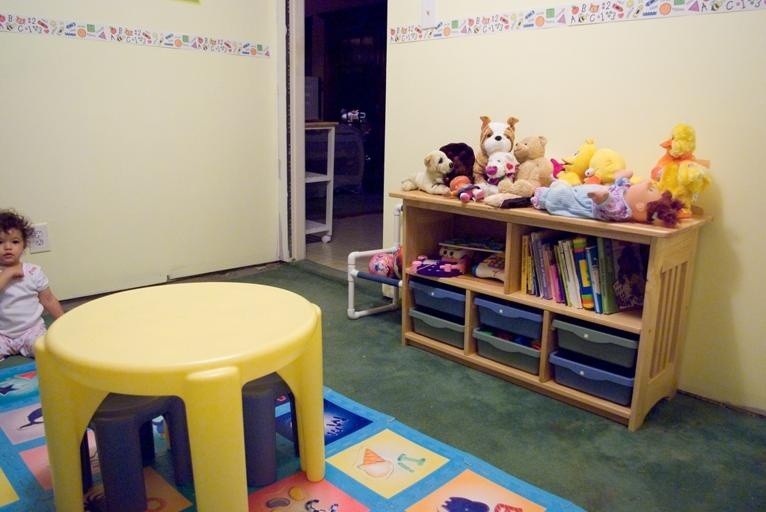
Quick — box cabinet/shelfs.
[390,190,707,432]
[305,120,341,245]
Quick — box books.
[520,229,646,315]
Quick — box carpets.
[0,355,584,512]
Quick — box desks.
[34,280,326,512]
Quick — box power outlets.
[25,224,54,257]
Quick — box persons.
[0,205,64,364]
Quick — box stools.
[242,372,300,487]
[79,393,194,509]
